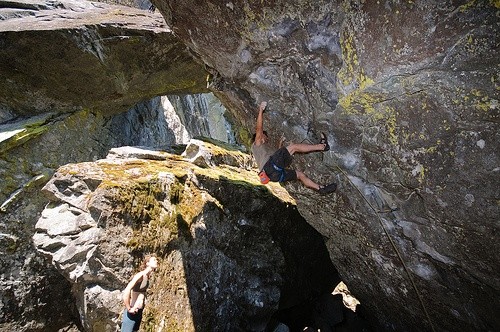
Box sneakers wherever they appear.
[318,183,337,195]
[320,132,330,151]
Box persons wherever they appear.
[249,99,338,196]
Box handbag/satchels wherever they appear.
[258,170,271,184]
